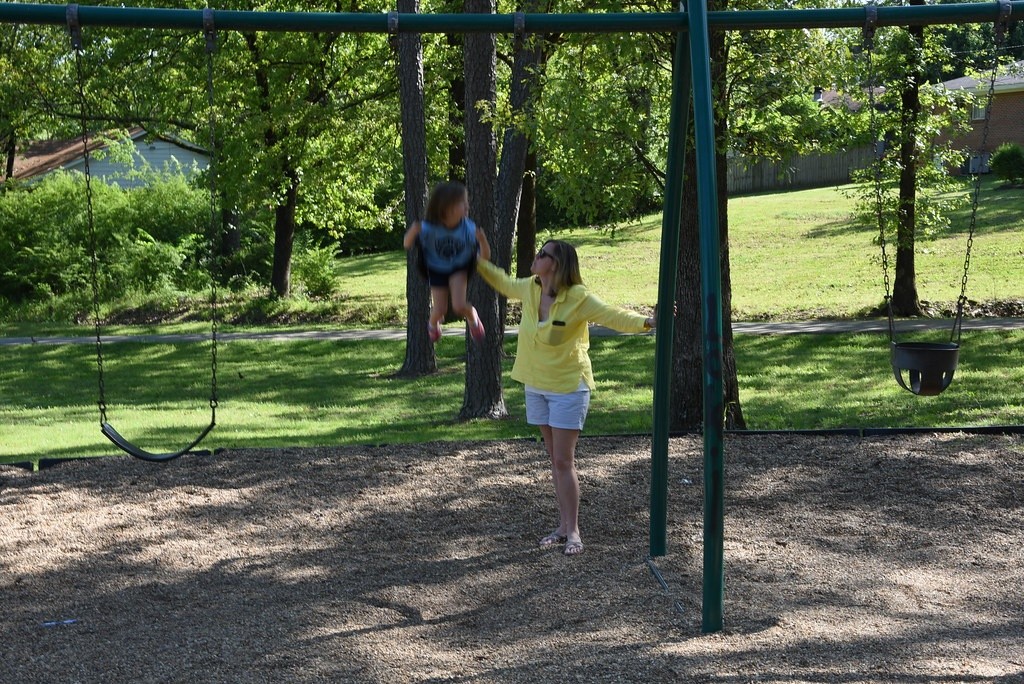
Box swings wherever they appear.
[387,36,528,294]
[72,34,222,463]
[860,21,1010,397]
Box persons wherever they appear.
[404,182,490,343]
[476,239,677,555]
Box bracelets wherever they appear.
[646,318,654,329]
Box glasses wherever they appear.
[539,249,555,263]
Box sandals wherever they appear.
[564,529,585,557]
[539,531,567,546]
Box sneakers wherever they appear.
[428,320,442,343]
[466,306,485,345]
